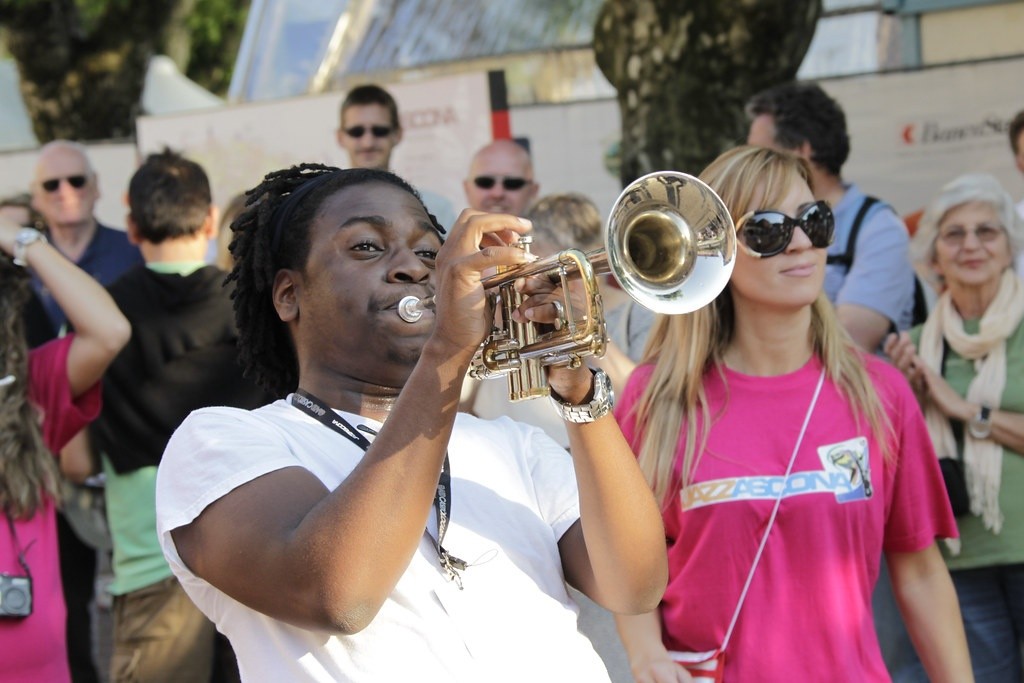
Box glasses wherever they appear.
[343,126,395,138]
[933,226,1004,246]
[731,201,837,259]
[470,176,532,191]
[42,176,90,192]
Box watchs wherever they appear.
[13,227,47,267]
[550,367,615,423]
[969,406,992,439]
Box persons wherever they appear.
[463,142,625,318]
[30,140,145,683]
[59,151,278,683]
[883,174,1024,683]
[156,163,669,683]
[615,144,974,683]
[1006,111,1024,279]
[339,85,403,171]
[744,84,915,362]
[0,207,132,683]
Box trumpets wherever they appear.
[398,170,738,407]
[489,192,603,258]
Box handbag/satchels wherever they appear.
[932,456,972,520]
